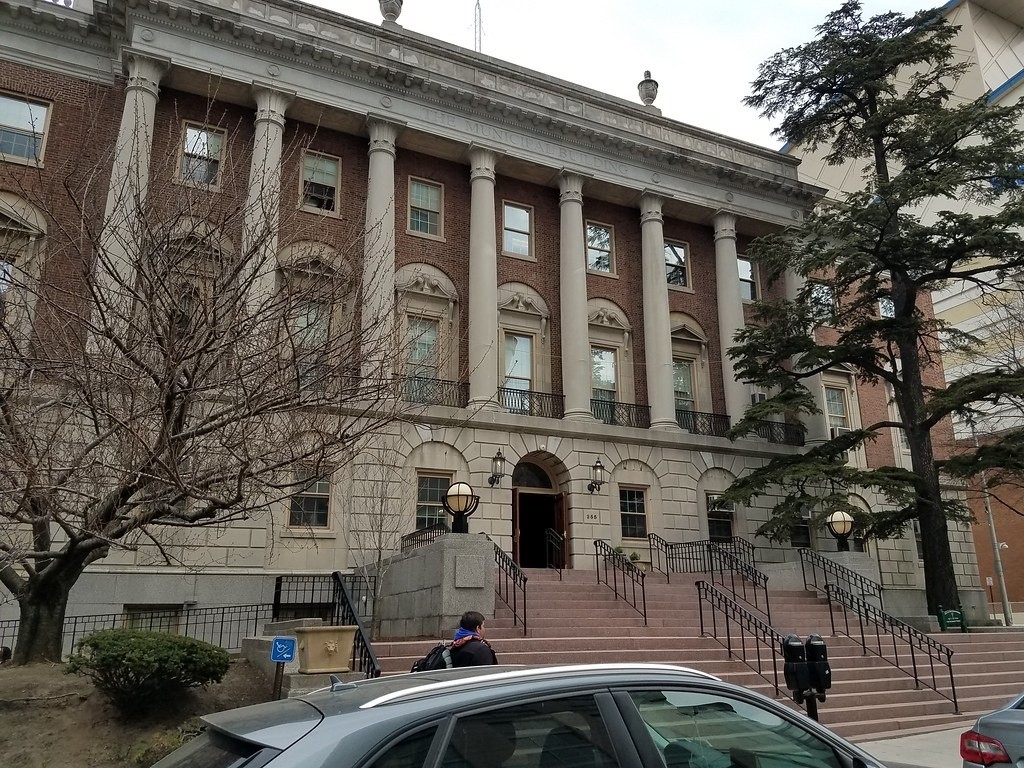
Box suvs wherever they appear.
[140,658,894,768]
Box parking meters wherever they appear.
[783,632,832,724]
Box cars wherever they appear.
[959,689,1024,768]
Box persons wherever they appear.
[449,611,499,667]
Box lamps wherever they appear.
[488,448,504,485]
[587,458,604,492]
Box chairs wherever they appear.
[542,727,598,768]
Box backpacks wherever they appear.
[411,639,482,673]
